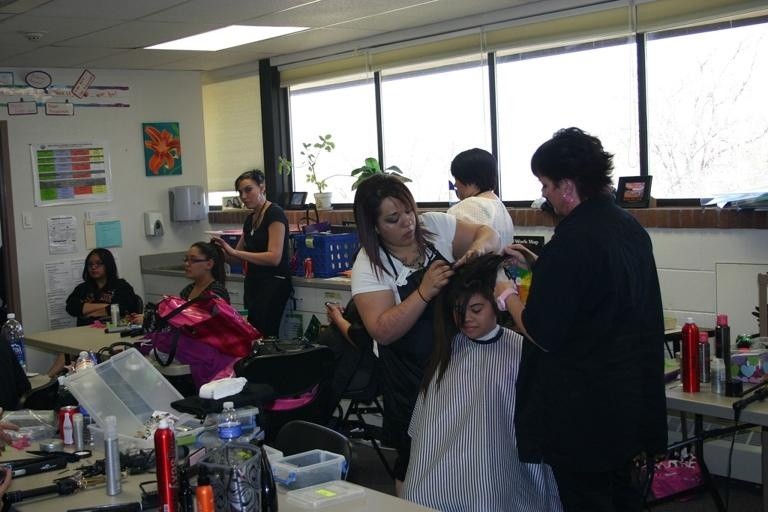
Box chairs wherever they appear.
[273,419,353,481]
[339,368,397,482]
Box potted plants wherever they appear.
[279,134,359,211]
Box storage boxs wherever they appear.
[62,348,197,438]
[89,412,206,460]
[286,481,365,512]
[270,449,347,491]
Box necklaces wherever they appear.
[250,201,267,236]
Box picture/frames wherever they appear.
[615,176,652,208]
[289,191,307,210]
[221,196,244,210]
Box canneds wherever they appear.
[241,260,247,276]
[59,405,80,444]
[304,258,314,279]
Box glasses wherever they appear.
[183,255,209,263]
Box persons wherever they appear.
[180,239,230,306]
[65,248,137,328]
[318,126,668,512]
[211,169,293,338]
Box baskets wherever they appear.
[220,234,249,273]
[289,224,359,280]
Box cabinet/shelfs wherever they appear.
[143,261,351,351]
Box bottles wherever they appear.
[216,401,239,442]
[714,312,732,396]
[62,412,73,444]
[710,355,725,397]
[101,415,122,496]
[194,467,214,511]
[2,312,26,373]
[73,411,84,454]
[153,419,180,512]
[79,381,97,446]
[680,316,701,395]
[696,330,711,385]
[109,303,121,328]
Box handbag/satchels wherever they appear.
[634,442,708,502]
[296,202,331,235]
[233,334,335,400]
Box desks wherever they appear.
[22,324,191,378]
[660,381,768,511]
[663,325,716,359]
